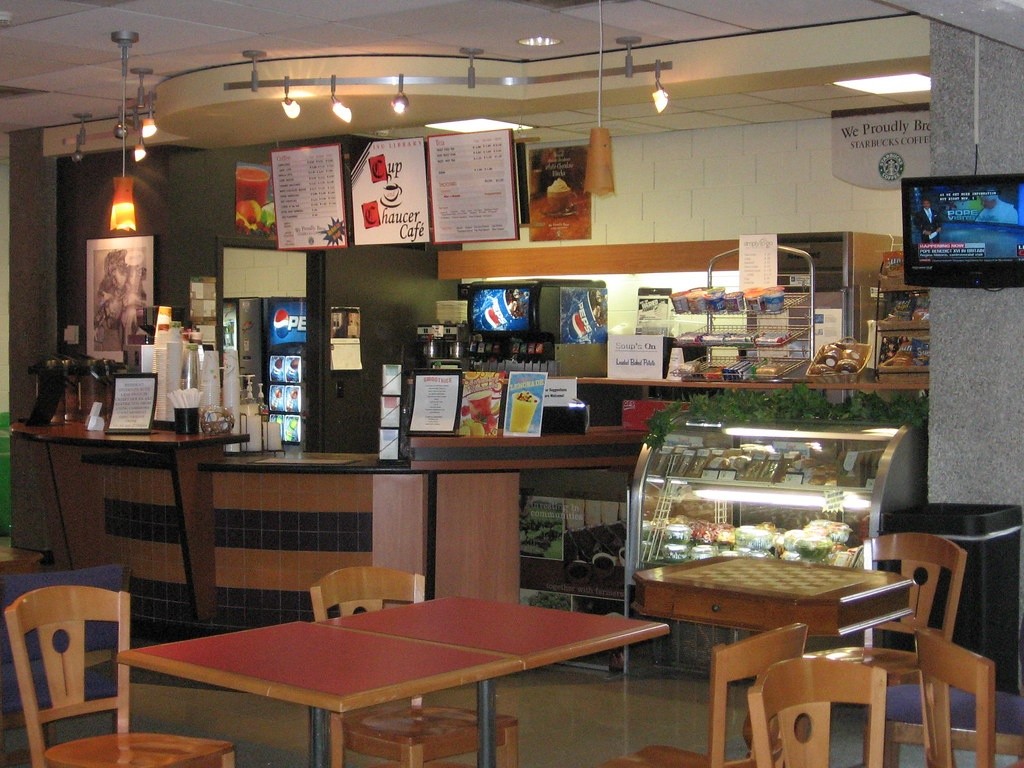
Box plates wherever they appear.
[540,208,577,216]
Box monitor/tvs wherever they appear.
[901,174,1024,289]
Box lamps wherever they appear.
[69,33,163,231]
[584,0,614,199]
[651,62,672,110]
[327,77,351,123]
[391,72,410,115]
[283,77,302,118]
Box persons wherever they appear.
[913,199,944,245]
[974,190,1018,224]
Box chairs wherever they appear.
[0,531,1024,768]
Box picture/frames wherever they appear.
[82,233,158,364]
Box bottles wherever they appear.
[286,418,298,441]
[275,415,282,423]
[271,387,283,410]
[287,358,300,381]
[271,357,284,379]
[287,388,298,411]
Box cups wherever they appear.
[481,290,515,330]
[152,306,241,452]
[174,407,197,433]
[619,547,625,566]
[666,348,684,381]
[566,560,591,585]
[509,393,540,433]
[561,291,603,343]
[236,166,270,207]
[592,552,618,581]
[546,187,577,210]
[467,392,492,417]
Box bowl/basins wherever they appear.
[670,287,788,312]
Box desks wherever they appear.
[633,554,916,766]
[115,619,522,767]
[315,596,670,768]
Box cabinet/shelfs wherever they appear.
[626,415,921,649]
[875,250,936,378]
[668,245,815,381]
